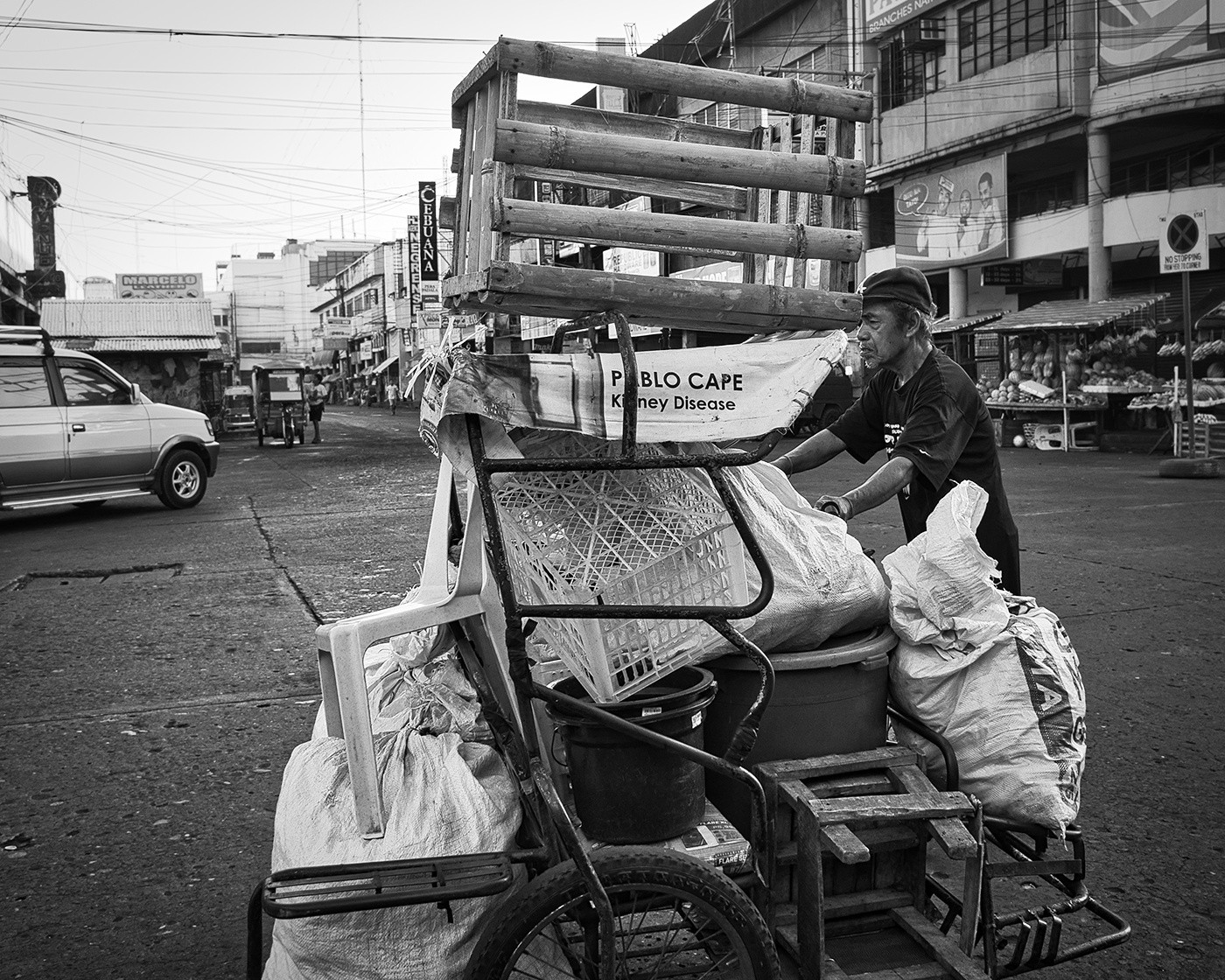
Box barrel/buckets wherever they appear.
[544,663,718,845]
[706,623,900,825]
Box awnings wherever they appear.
[36,337,222,352]
[373,355,399,374]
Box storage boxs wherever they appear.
[1081,384,1127,394]
[1146,385,1162,392]
[498,432,756,704]
[1128,388,1148,393]
[992,418,1026,448]
[1019,380,1055,398]
[1177,421,1225,458]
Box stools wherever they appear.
[747,744,993,980]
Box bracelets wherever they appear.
[842,496,853,519]
[784,455,792,479]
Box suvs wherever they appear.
[0,323,223,517]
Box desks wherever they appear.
[1126,398,1225,455]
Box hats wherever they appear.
[852,267,933,317]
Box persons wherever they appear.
[769,266,1021,615]
[386,379,399,415]
[916,171,1002,257]
[308,374,327,443]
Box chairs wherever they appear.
[1033,421,1100,452]
[312,446,557,841]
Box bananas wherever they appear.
[1157,339,1225,361]
[1068,326,1157,361]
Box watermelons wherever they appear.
[1193,362,1225,401]
[1008,340,1133,388]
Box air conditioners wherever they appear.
[899,17,945,51]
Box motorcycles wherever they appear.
[251,359,310,450]
